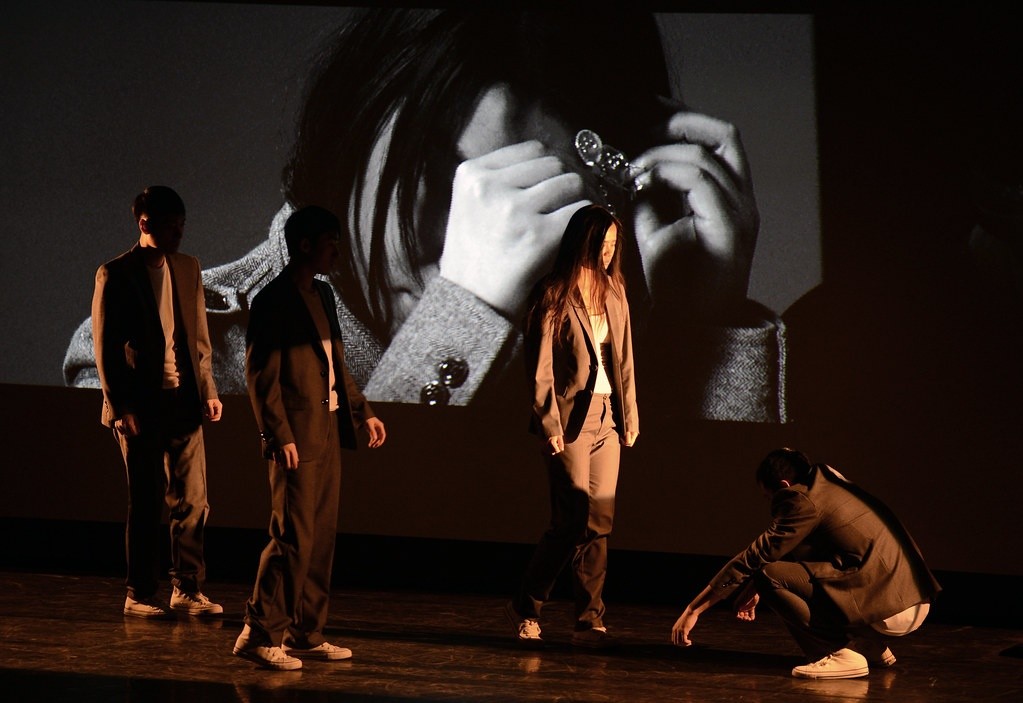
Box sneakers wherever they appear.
[506,599,544,643]
[124,595,169,617]
[847,640,896,668]
[170,586,223,615]
[281,637,352,660]
[791,647,870,679]
[233,622,302,671]
[570,627,625,650]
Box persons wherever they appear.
[92,185,223,618]
[229,206,386,671]
[670,448,941,679]
[61,0,794,425]
[505,205,639,641]
